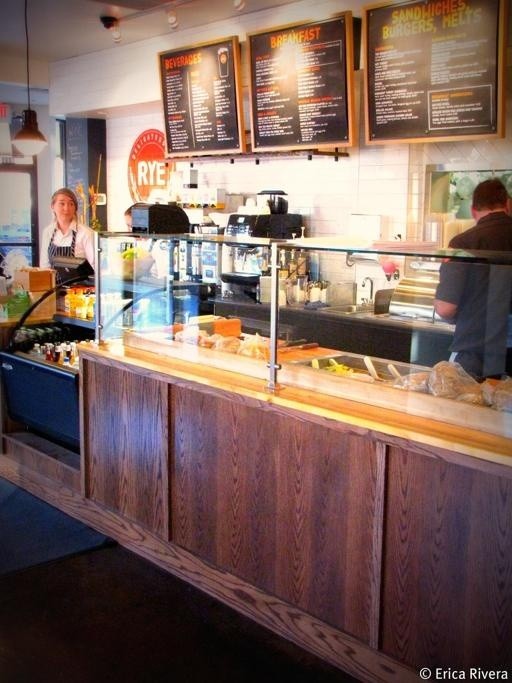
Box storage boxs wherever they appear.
[0,270,55,317]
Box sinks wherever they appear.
[317,304,374,316]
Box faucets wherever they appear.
[362,277,373,304]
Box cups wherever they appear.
[287,275,308,306]
[217,48,230,78]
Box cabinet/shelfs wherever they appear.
[0,318,59,432]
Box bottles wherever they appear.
[14,287,96,368]
[261,248,307,278]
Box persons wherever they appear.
[40,187,95,271]
[124,203,146,232]
[433,179,512,384]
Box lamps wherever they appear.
[110,29,122,46]
[167,10,178,29]
[10,0,49,156]
[233,2,247,11]
